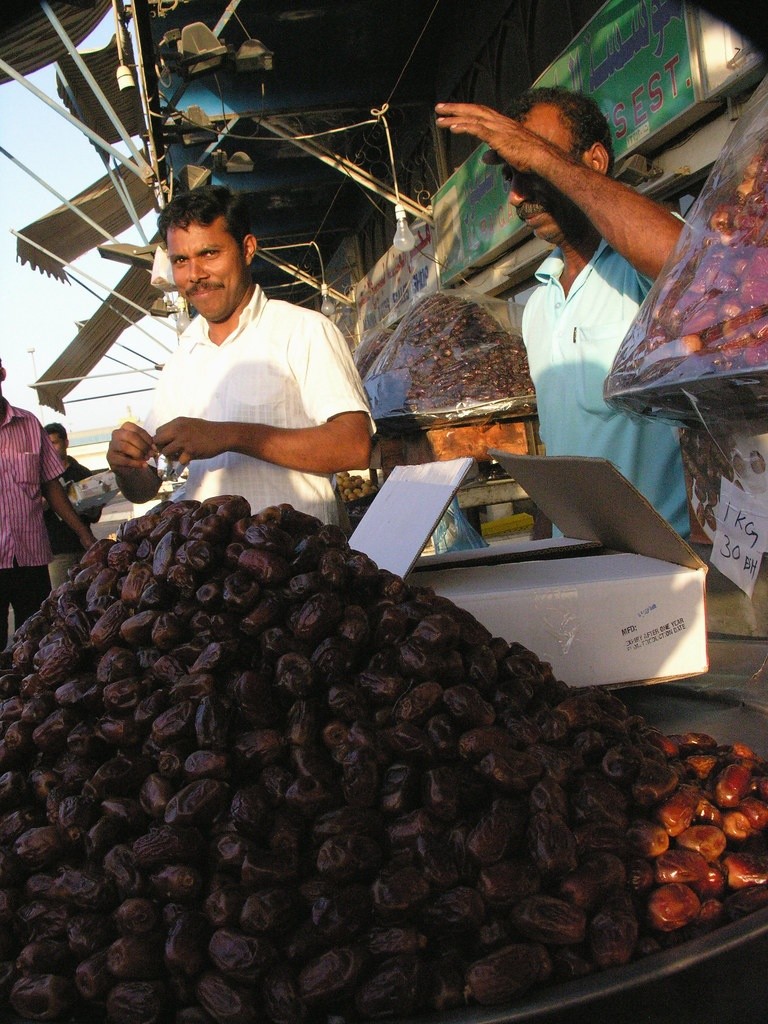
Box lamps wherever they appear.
[176,21,227,83]
[177,163,211,191]
[112,0,136,93]
[150,297,169,318]
[179,104,220,148]
[226,151,254,173]
[97,243,154,271]
[234,38,274,74]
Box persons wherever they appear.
[108,184,381,531]
[0,358,101,657]
[40,422,103,590]
[432,86,688,542]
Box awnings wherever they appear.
[55,33,146,151]
[0,0,159,188]
[12,149,181,357]
[28,225,171,416]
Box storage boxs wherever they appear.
[54,470,120,521]
[347,449,710,691]
